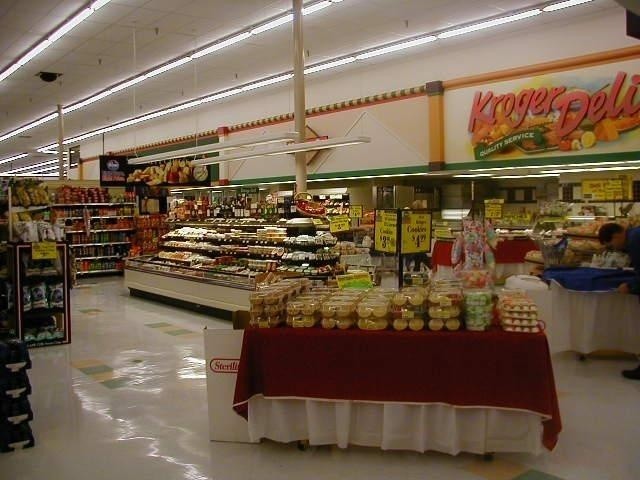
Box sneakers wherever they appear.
[621,366,640,379]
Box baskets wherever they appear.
[296,199,328,218]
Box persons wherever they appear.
[598,224,640,380]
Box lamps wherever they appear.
[190,30,371,167]
[126,20,300,165]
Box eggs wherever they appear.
[499,292,539,332]
